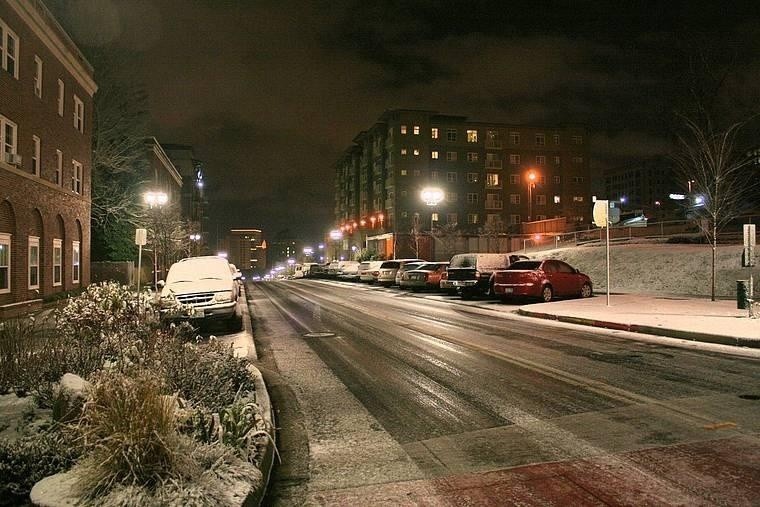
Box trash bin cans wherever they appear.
[746,297,760,318]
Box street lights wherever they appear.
[330,228,344,261]
[143,189,169,295]
[526,168,539,221]
[190,232,202,256]
[419,185,446,261]
[688,178,696,194]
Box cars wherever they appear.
[154,253,246,335]
